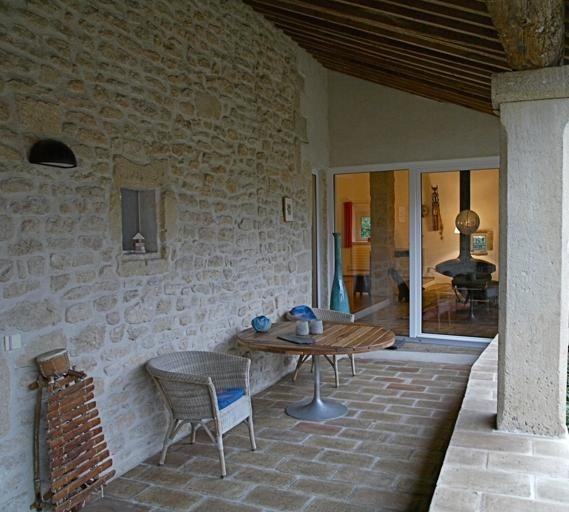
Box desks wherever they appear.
[235,319,396,422]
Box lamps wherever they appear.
[29,138,76,169]
[455,171,480,236]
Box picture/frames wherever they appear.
[283,196,294,223]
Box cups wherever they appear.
[295,320,324,336]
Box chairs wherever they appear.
[285,307,357,388]
[143,350,256,478]
